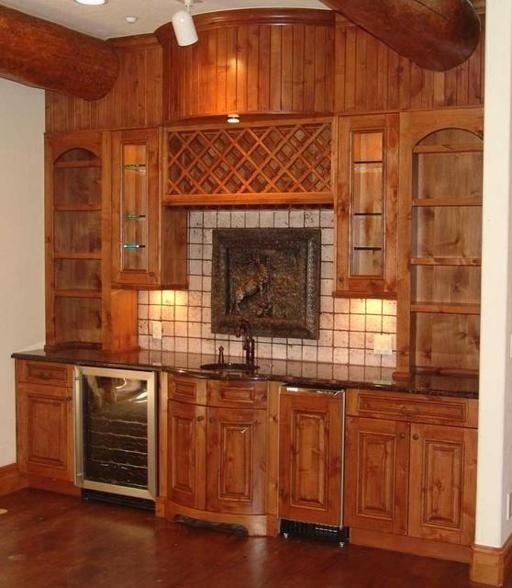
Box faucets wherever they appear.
[235,319,255,367]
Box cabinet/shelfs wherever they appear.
[161,120,334,205]
[157,371,274,536]
[347,388,479,564]
[44,135,138,353]
[14,356,79,496]
[112,128,187,290]
[392,106,486,380]
[275,385,346,531]
[334,113,399,300]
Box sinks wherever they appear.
[200,363,259,370]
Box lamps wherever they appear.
[171,0,202,47]
